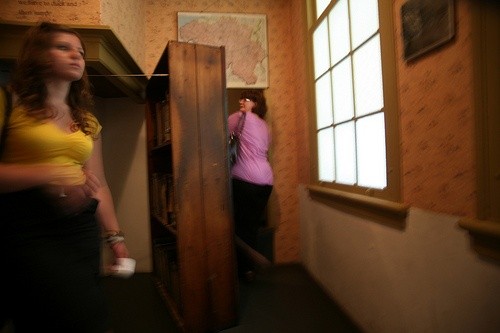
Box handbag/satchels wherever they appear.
[229,112,247,163]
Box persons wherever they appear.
[227,91,273,287]
[0,23,129,333]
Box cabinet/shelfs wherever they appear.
[145,40,242,333]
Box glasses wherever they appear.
[238,98,252,102]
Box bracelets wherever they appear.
[105,232,126,248]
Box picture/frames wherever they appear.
[399,0,458,66]
